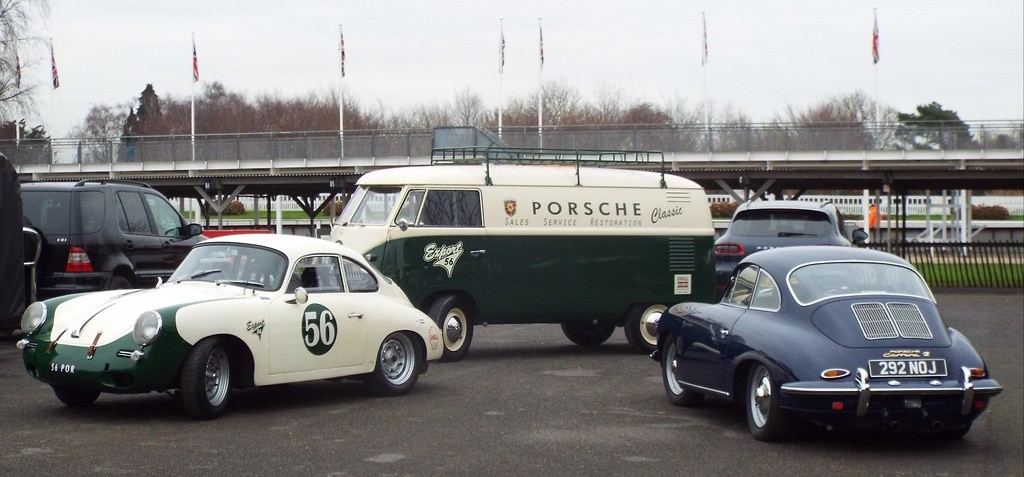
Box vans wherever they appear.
[323,144,717,362]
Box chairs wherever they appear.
[750,213,772,235]
[801,220,828,235]
[300,267,329,287]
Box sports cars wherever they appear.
[649,243,1005,441]
[16,231,446,419]
[714,198,872,301]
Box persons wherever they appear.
[869,203,883,242]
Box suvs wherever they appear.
[19,176,211,302]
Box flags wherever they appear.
[872,8,880,64]
[537,18,545,67]
[339,26,346,80]
[50,35,59,90]
[702,11,709,66]
[192,32,199,85]
[499,20,507,73]
